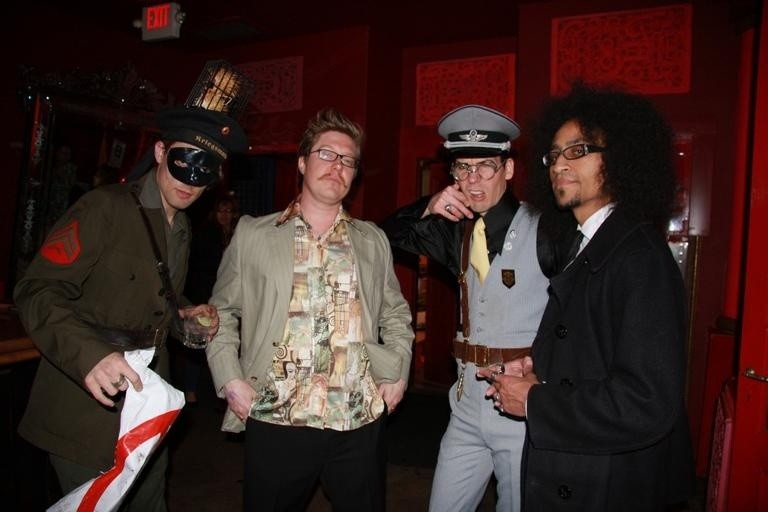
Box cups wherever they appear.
[183,307,214,349]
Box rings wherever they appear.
[496,392,500,401]
[496,363,505,375]
[110,374,126,389]
[490,371,497,381]
[444,204,453,212]
[499,403,504,413]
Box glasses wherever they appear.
[448,159,506,181]
[541,143,604,167]
[308,148,360,169]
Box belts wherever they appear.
[452,340,532,367]
[80,319,173,349]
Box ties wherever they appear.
[564,230,584,267]
[468,217,490,282]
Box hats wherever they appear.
[436,103,521,156]
[153,107,246,163]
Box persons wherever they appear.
[373,102,550,512]
[201,111,416,512]
[474,75,700,511]
[12,105,253,511]
[166,192,245,442]
